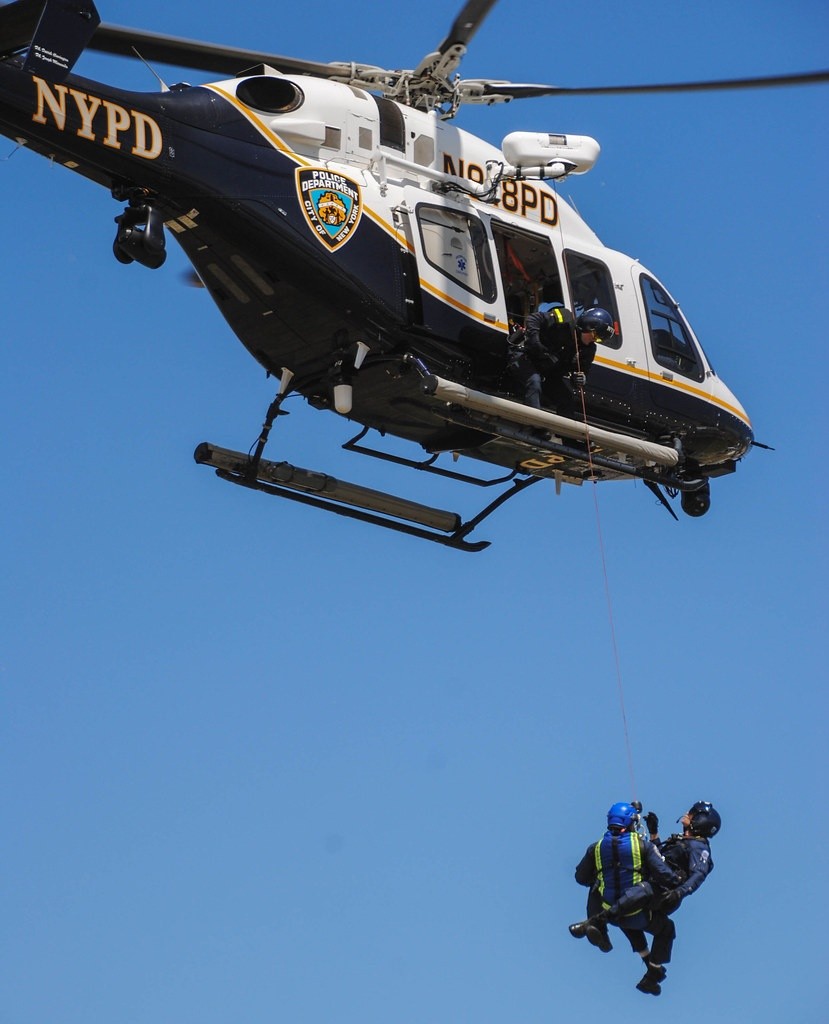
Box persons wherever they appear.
[568,800,721,996]
[509,302,616,410]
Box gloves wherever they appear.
[643,811,658,834]
[659,885,679,909]
[570,372,586,386]
[536,343,549,361]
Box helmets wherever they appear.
[607,803,638,830]
[577,308,614,340]
[692,802,721,838]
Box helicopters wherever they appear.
[0,1,829,555]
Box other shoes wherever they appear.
[658,966,667,982]
[636,976,661,996]
[586,925,613,952]
[569,920,592,938]
